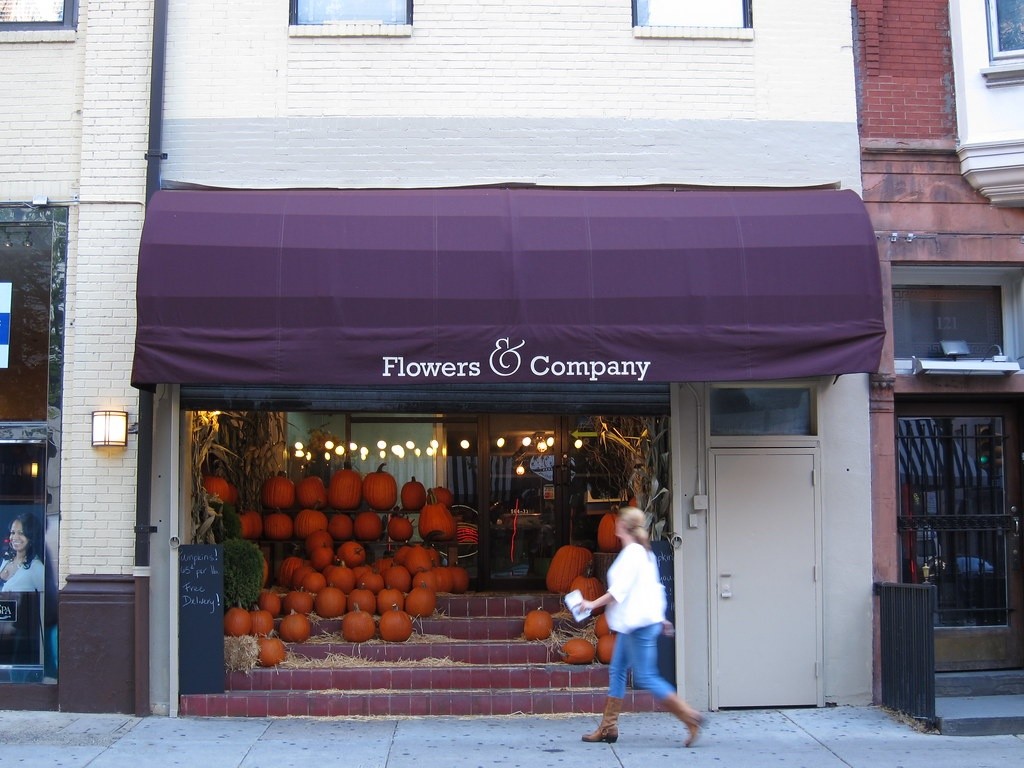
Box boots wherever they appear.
[662,693,707,747]
[582,696,623,742]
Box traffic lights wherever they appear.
[992,431,1005,468]
[977,424,994,469]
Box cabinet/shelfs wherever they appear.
[256,507,461,577]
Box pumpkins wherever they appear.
[557,613,617,664]
[204,460,469,666]
[545,542,605,617]
[524,606,554,641]
[597,510,623,553]
[630,497,636,507]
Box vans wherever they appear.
[915,529,941,565]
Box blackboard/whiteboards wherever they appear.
[178,545,226,704]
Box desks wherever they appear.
[502,513,543,557]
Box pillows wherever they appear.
[456,528,478,543]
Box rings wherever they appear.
[582,606,584,608]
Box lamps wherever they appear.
[980,343,1008,363]
[942,339,971,361]
[91,411,128,446]
[31,463,38,478]
[534,432,548,452]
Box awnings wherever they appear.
[130,186,887,384]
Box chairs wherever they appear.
[449,505,480,559]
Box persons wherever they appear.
[0,512,44,592]
[580,507,706,748]
